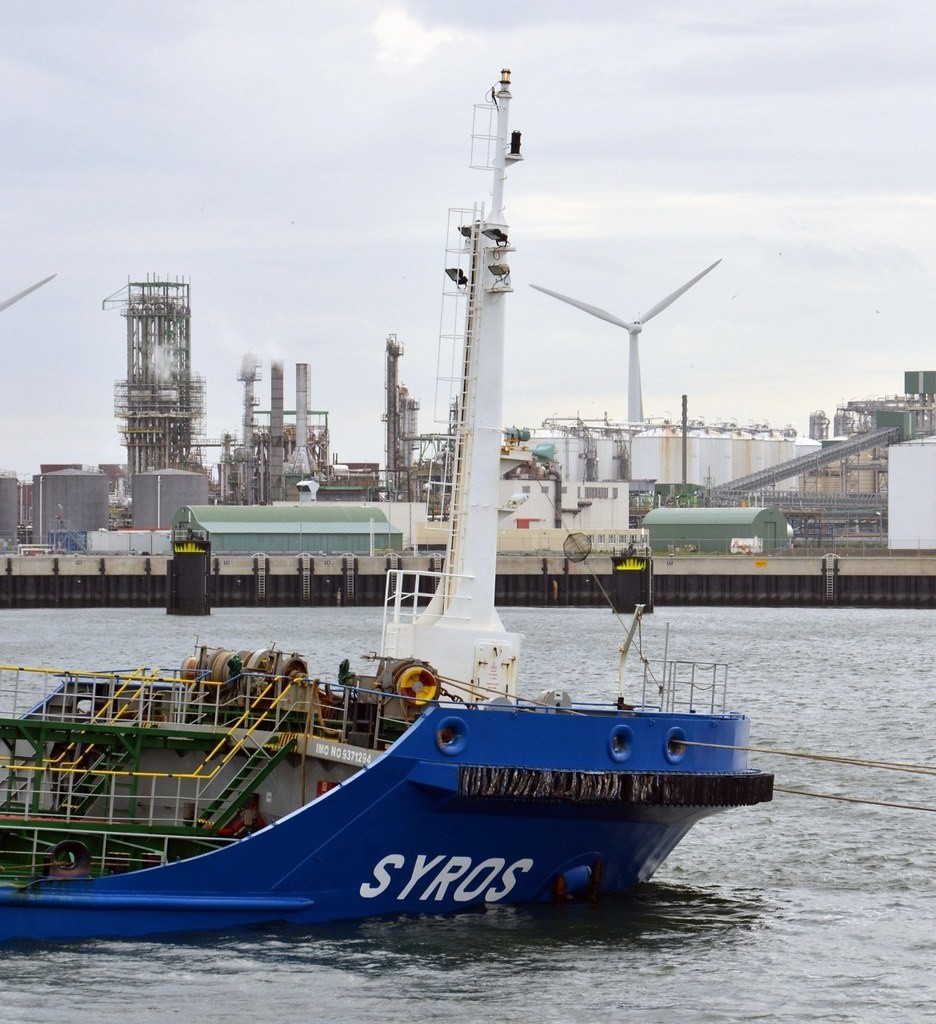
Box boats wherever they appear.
[0,70,775,945]
